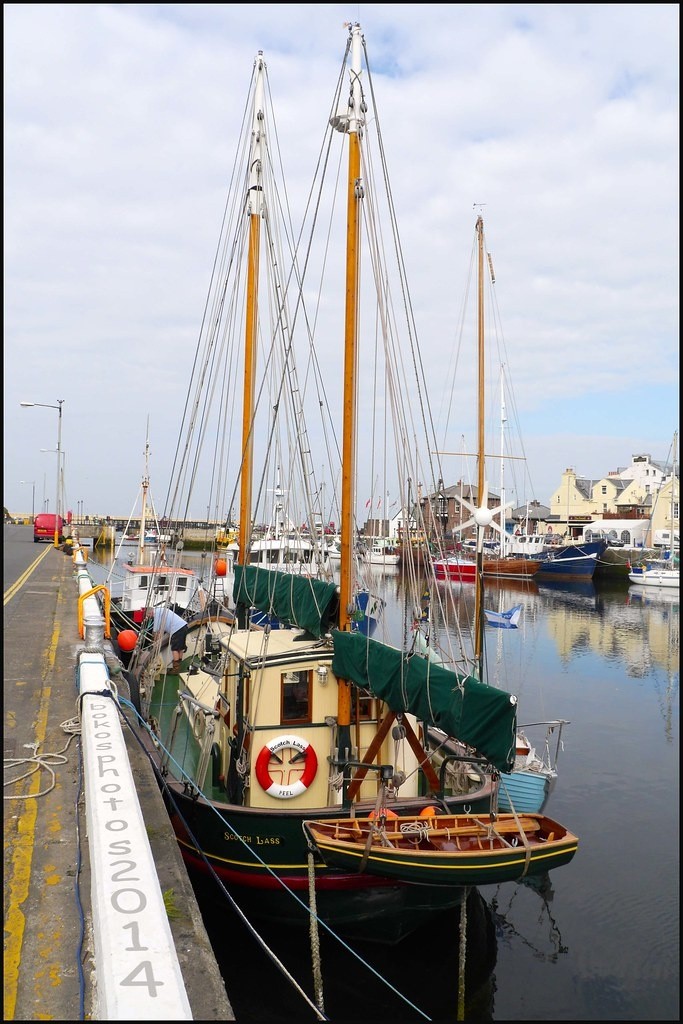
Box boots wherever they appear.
[167,658,182,675]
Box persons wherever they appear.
[145,606,189,675]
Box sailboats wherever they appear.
[86,19,683,894]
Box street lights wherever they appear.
[20,399,64,549]
[40,447,64,528]
[19,480,35,524]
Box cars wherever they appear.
[33,513,66,544]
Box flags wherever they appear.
[484,605,522,629]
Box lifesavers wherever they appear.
[256,735,320,800]
